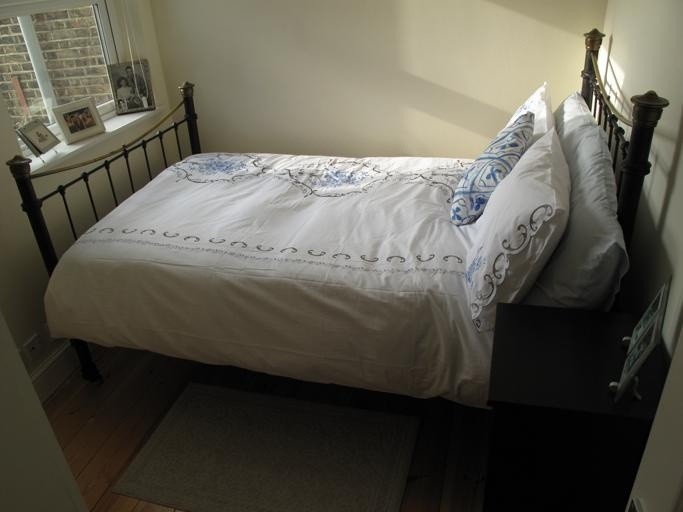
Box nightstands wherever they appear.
[486,299,672,512]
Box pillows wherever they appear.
[461,123,574,337]
[451,108,535,227]
[496,80,555,143]
[541,123,629,308]
[554,88,598,144]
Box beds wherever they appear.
[7,28,670,397]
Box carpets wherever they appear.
[112,381,422,512]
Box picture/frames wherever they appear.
[606,285,670,396]
[16,56,156,156]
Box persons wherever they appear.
[116,77,132,99]
[118,98,127,111]
[128,97,143,109]
[125,65,151,106]
